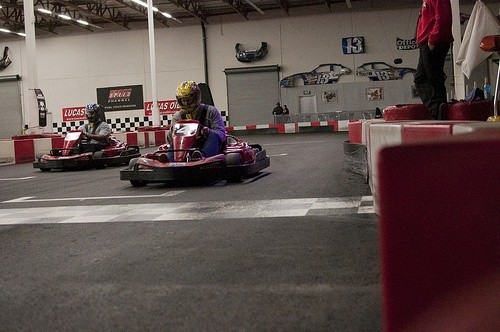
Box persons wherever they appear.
[159,81,227,161]
[272,102,283,115]
[52,104,110,156]
[414,0,454,120]
[282,105,289,116]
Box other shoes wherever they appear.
[159,154,169,163]
[52,148,60,156]
[192,150,202,161]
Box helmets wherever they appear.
[175,81,202,114]
[85,103,100,123]
